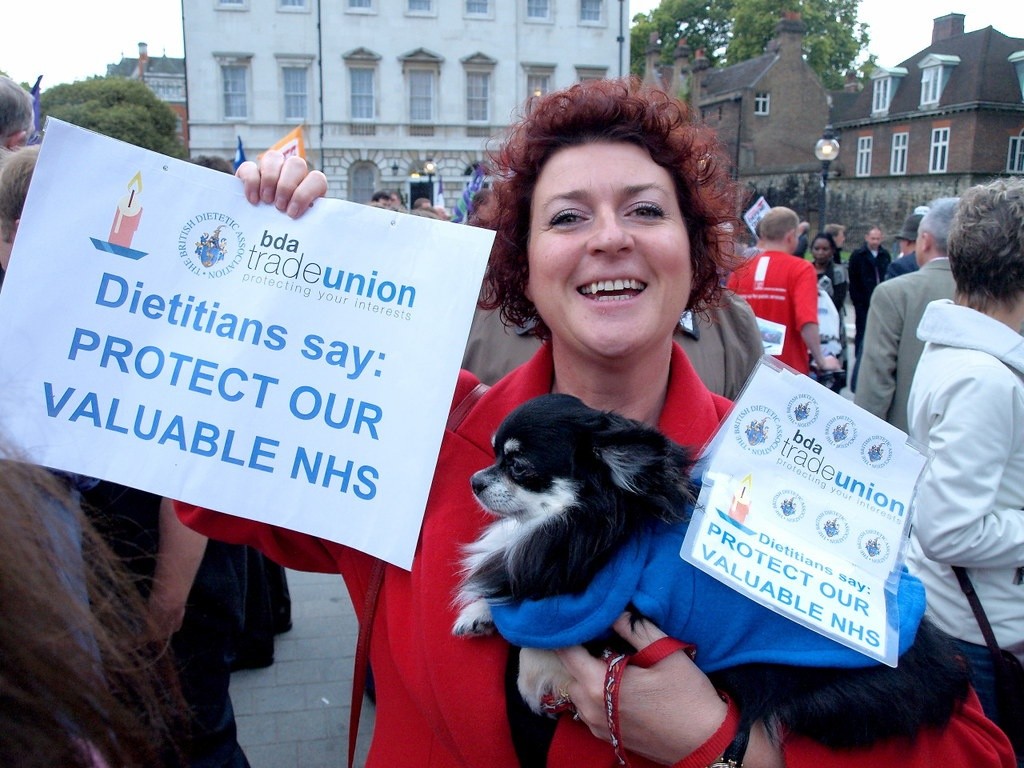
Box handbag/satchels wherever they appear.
[992,648,1024,763]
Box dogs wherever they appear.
[449,391,973,755]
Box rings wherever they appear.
[559,677,573,704]
[572,712,582,722]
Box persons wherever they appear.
[847,225,893,395]
[0,148,257,767]
[724,207,844,378]
[885,212,926,285]
[168,74,1017,768]
[454,277,760,402]
[716,217,845,335]
[904,175,1023,768]
[2,73,37,150]
[856,195,963,438]
[362,186,501,228]
[181,151,300,671]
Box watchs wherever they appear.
[706,707,748,768]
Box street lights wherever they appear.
[812,123,840,238]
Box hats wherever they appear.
[893,214,924,242]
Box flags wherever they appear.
[231,135,249,170]
[258,120,307,161]
[26,75,44,145]
[453,163,484,223]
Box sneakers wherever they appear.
[223,650,275,672]
